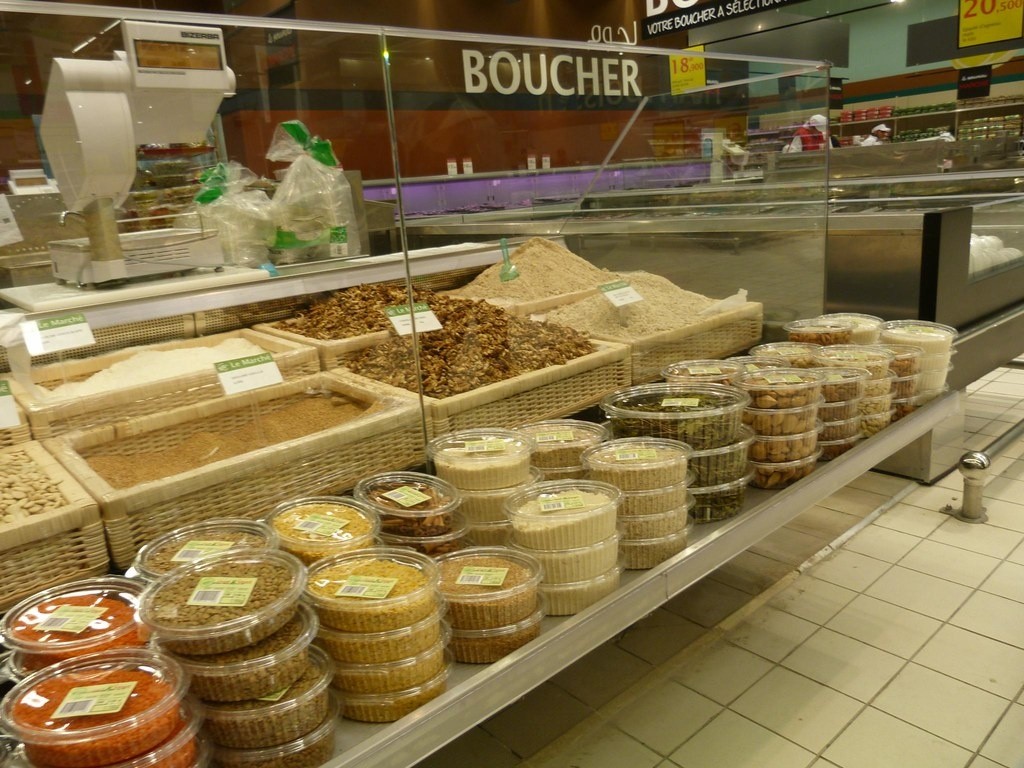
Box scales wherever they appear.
[722,138,763,179]
[38,19,237,288]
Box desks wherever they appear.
[0,265,268,312]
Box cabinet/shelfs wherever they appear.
[741,96,1024,169]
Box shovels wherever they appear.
[500,238,520,281]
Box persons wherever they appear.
[861,124,892,146]
[782,115,833,152]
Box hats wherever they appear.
[810,115,827,127]
[872,124,891,133]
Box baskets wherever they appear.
[0,272,762,601]
